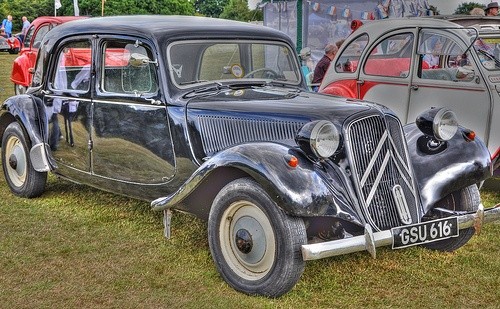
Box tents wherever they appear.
[258,0,438,92]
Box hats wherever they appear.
[484,2,500,11]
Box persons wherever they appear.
[1,15,13,38]
[22,16,30,36]
[298,3,500,79]
[312,42,338,92]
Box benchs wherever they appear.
[346,58,430,76]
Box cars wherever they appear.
[317,16,500,171]
[10,15,132,96]
[0,13,500,299]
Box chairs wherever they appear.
[171,47,190,84]
